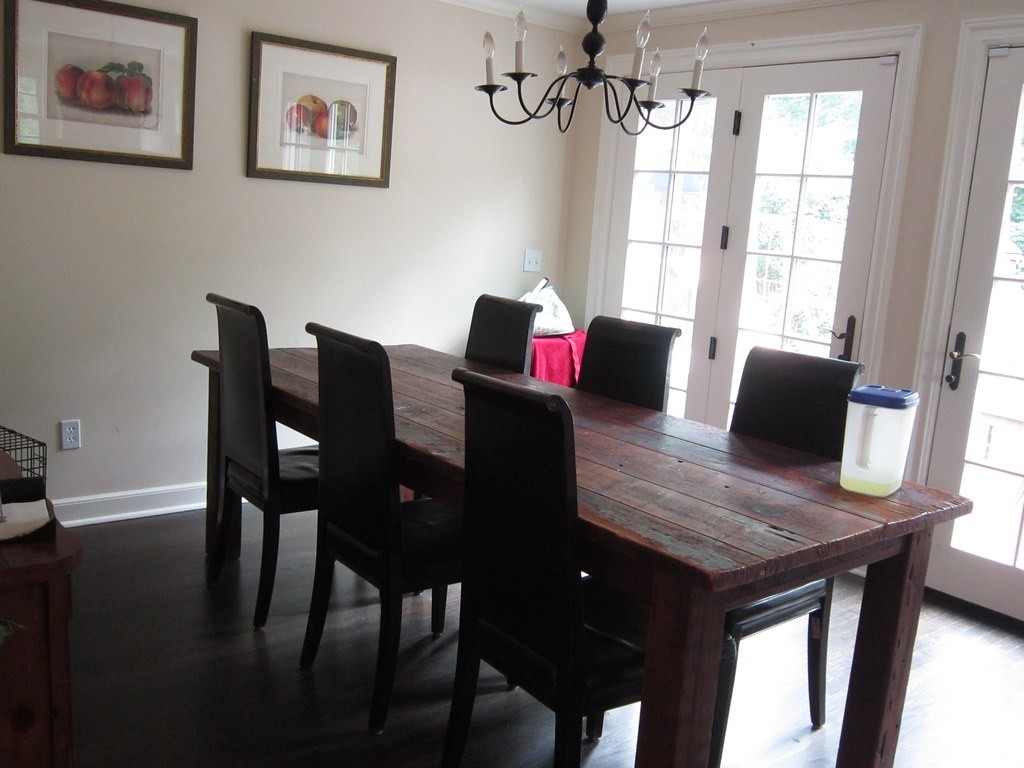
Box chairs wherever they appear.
[440,369,739,768]
[464,294,543,376]
[299,321,462,736]
[575,315,682,414]
[205,293,322,630]
[724,346,866,727]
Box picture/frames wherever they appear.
[4,0,199,169]
[246,31,398,189]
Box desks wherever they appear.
[191,343,975,767]
[0,516,85,768]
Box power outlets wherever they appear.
[60,419,81,450]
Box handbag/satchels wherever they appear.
[520,278,575,336]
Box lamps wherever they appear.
[474,0,713,135]
